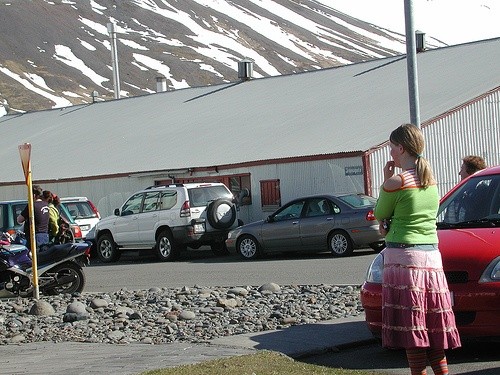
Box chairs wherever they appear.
[308,203,322,216]
[322,200,335,214]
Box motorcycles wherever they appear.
[0,224,93,298]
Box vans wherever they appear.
[0,197,101,240]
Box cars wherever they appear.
[359,164,500,337]
[224,192,388,260]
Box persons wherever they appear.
[458,155,490,222]
[17,186,49,249]
[373,123,461,375]
[42,190,60,244]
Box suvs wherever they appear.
[96,182,239,260]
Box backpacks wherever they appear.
[49,207,75,244]
